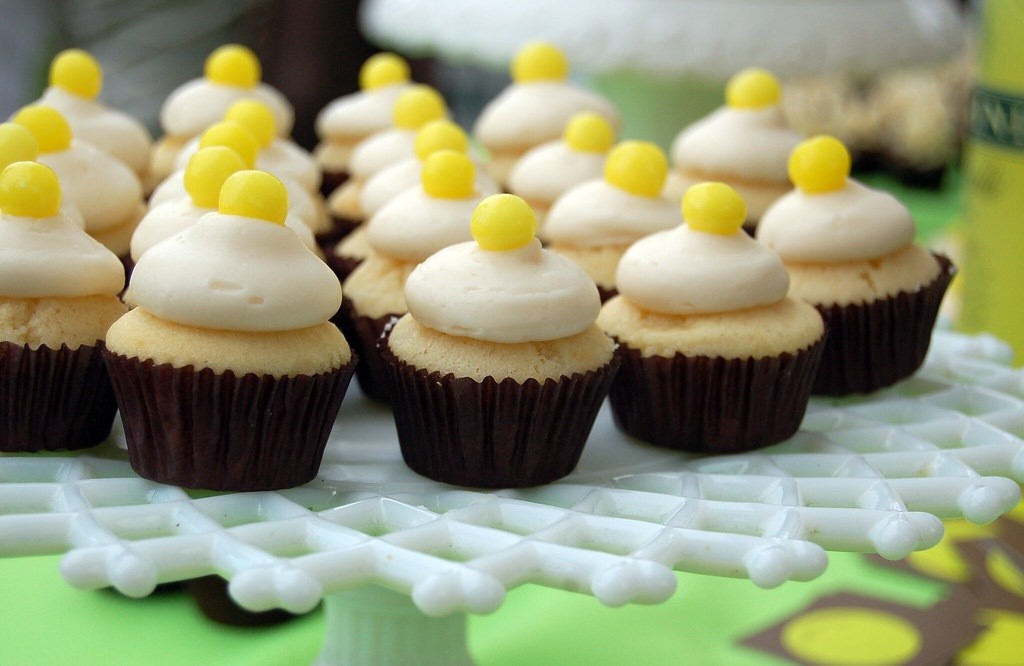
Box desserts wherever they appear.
[0,48,955,489]
